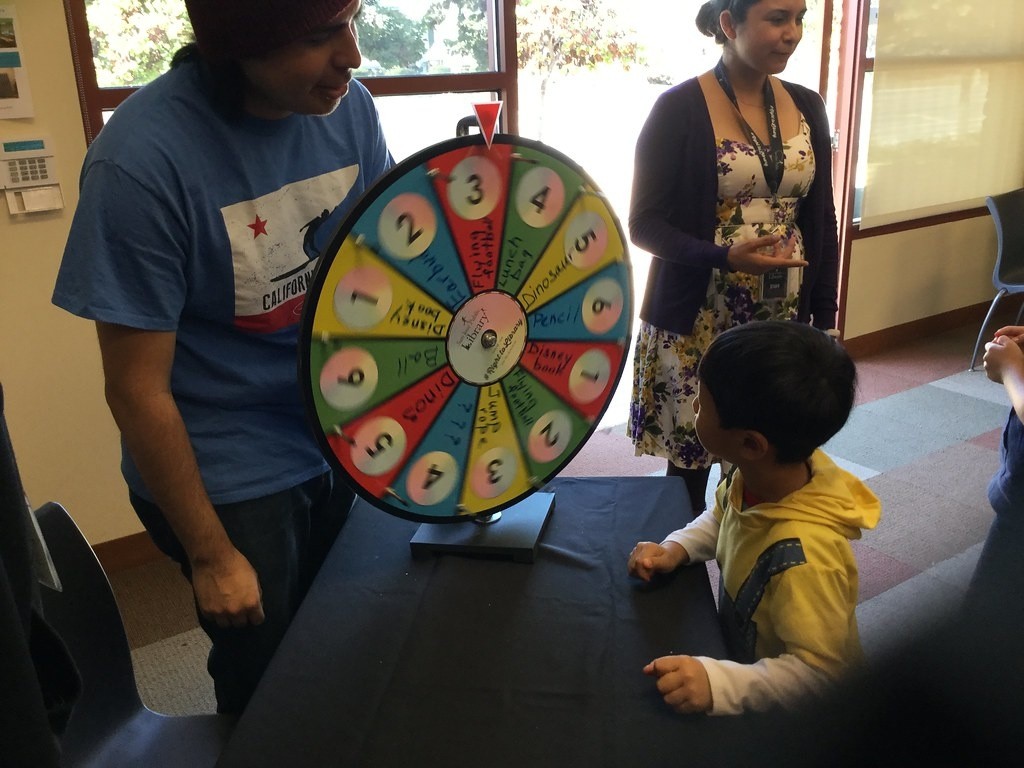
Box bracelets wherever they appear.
[822,328,841,339]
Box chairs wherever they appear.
[968,187,1024,373]
[0,499,241,767]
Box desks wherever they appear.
[217,475,748,768]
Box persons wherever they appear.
[962,325,1023,635]
[47,0,403,744]
[628,0,844,518]
[627,318,884,721]
